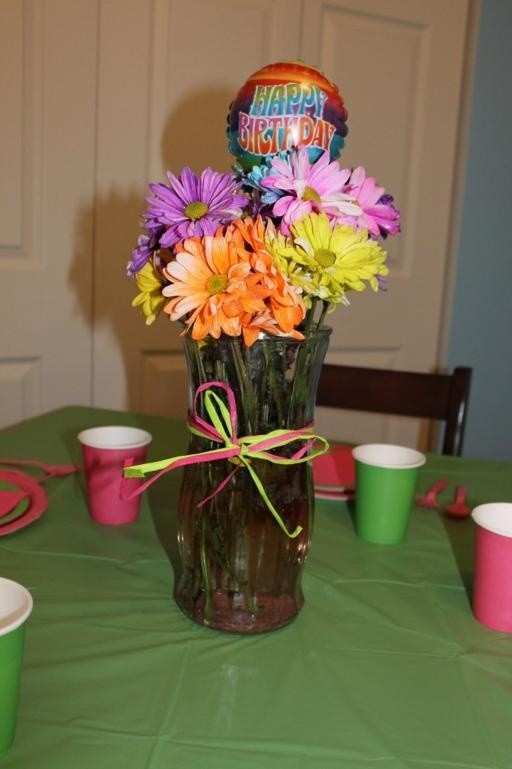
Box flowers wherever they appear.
[124,144,402,628]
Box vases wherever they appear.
[173,325,334,636]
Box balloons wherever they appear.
[225,61,349,167]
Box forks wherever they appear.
[415,478,449,510]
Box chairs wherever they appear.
[317,365,473,457]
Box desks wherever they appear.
[1,405,512,768]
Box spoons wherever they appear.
[445,484,472,519]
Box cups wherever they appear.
[468,500,512,636]
[0,575,35,762]
[76,425,155,527]
[351,442,428,548]
